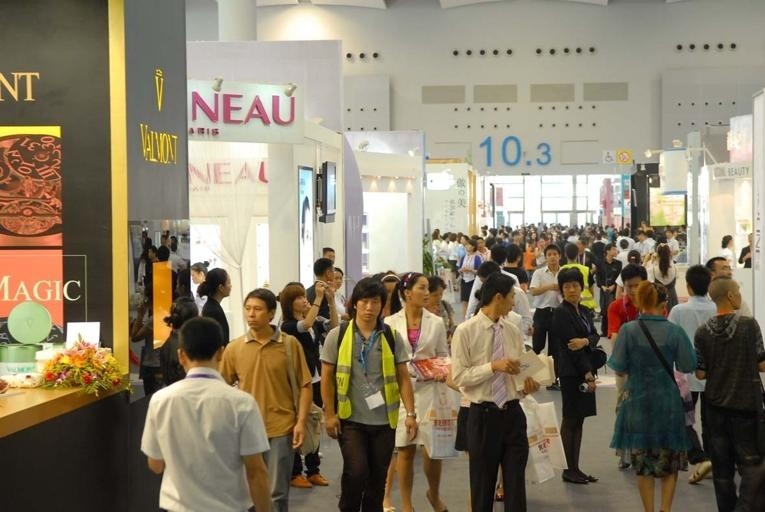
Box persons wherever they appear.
[602,264,669,390]
[323,247,335,265]
[595,243,622,337]
[705,257,753,316]
[669,265,720,483]
[475,262,535,501]
[440,232,449,264]
[275,282,305,302]
[547,265,607,485]
[530,243,566,391]
[694,274,765,512]
[451,273,542,512]
[333,266,351,322]
[423,274,458,344]
[479,221,553,249]
[191,261,209,286]
[632,230,655,255]
[159,297,201,385]
[615,250,642,298]
[320,277,418,512]
[575,234,599,296]
[432,230,442,258]
[739,232,753,268]
[219,288,334,512]
[554,221,633,252]
[377,272,401,512]
[139,318,274,511]
[616,229,635,252]
[719,234,735,269]
[666,230,681,263]
[276,283,338,488]
[476,237,492,261]
[197,269,233,358]
[448,233,459,269]
[465,243,521,322]
[384,272,451,509]
[646,242,678,311]
[564,242,598,319]
[606,279,699,512]
[134,230,190,393]
[455,235,469,266]
[302,258,337,345]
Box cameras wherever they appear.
[315,280,328,289]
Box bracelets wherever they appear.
[585,376,593,382]
[328,303,336,309]
[313,302,321,309]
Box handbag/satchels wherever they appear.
[637,318,696,428]
[522,397,568,485]
[282,333,327,457]
[418,378,461,459]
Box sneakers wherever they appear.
[688,460,712,484]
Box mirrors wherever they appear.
[127,219,191,406]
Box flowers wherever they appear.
[40,341,135,398]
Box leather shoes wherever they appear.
[580,472,599,482]
[425,489,449,512]
[383,504,396,512]
[308,473,330,486]
[289,476,313,488]
[496,487,504,501]
[562,470,589,484]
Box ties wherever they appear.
[490,324,508,409]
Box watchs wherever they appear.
[405,412,417,417]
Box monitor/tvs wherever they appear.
[314,162,337,224]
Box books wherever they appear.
[409,358,452,380]
